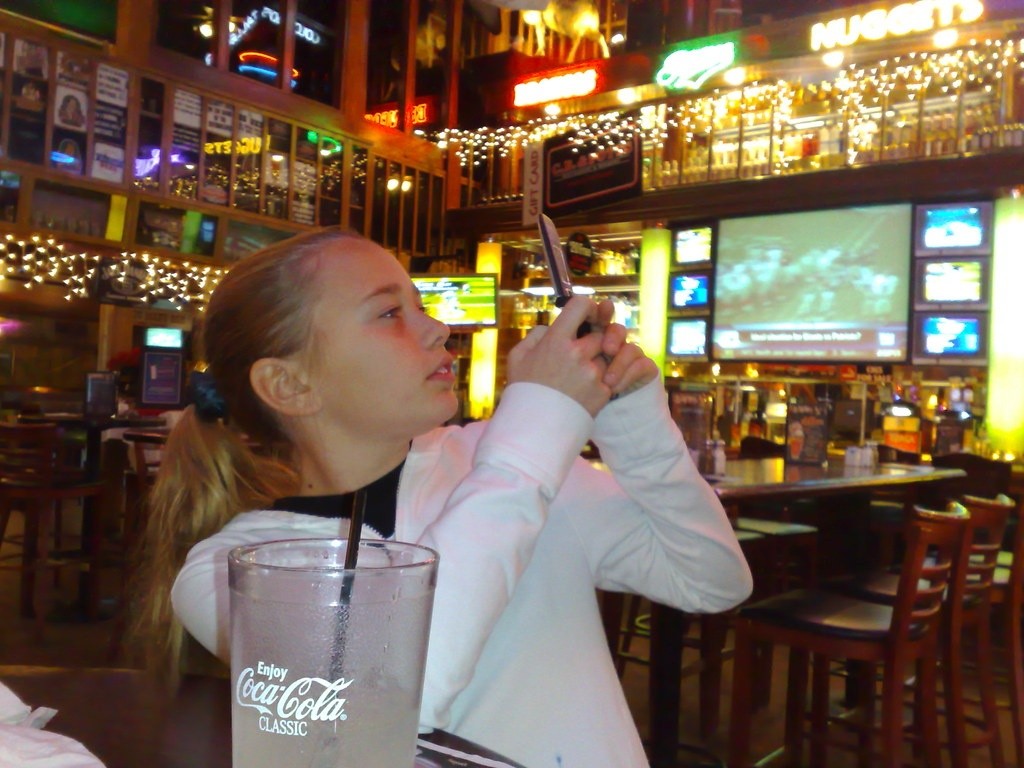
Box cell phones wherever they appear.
[537,213,591,340]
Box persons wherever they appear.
[137,226,753,768]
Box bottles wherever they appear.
[881,384,920,464]
[717,385,833,463]
[699,439,727,482]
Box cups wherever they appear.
[228,539,439,768]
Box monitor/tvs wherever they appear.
[662,199,988,366]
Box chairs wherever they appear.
[0,395,1024,768]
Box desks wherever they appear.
[0,670,527,768]
[602,448,969,768]
[16,415,167,624]
